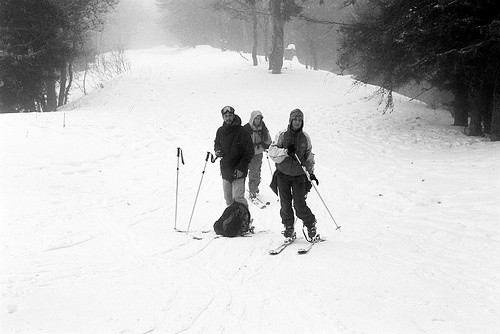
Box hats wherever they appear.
[289,108,304,126]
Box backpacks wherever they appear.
[214,202,248,238]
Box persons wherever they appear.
[268,109,319,241]
[243,110,272,202]
[214,106,255,208]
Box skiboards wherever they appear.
[245,189,270,209]
[269,234,321,254]
[193,218,271,240]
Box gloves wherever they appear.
[288,144,296,155]
[310,174,318,185]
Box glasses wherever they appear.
[221,106,234,114]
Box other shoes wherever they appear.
[285,225,294,238]
[249,189,258,198]
[307,223,316,237]
